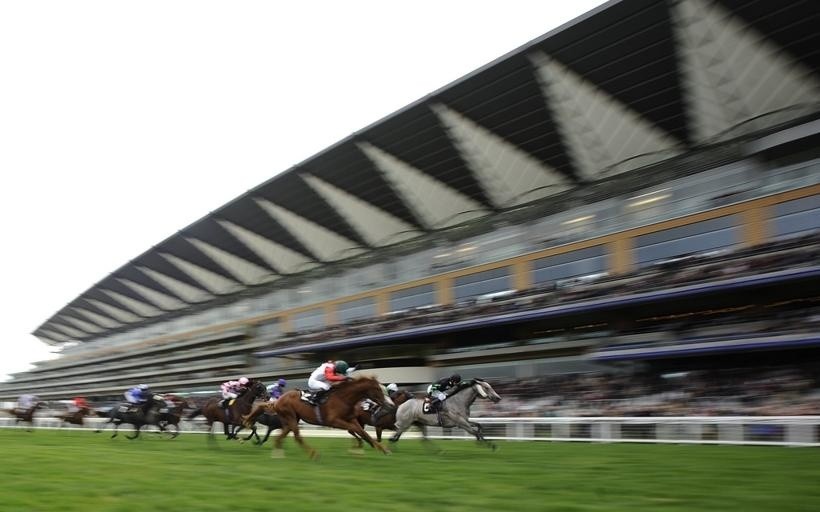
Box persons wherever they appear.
[219,377,252,408]
[265,378,288,401]
[421,374,464,415]
[365,381,398,415]
[305,359,355,406]
[122,383,151,409]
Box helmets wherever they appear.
[279,379,286,387]
[334,361,348,374]
[386,384,398,392]
[239,377,249,385]
[451,375,461,383]
[140,384,148,390]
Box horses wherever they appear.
[388,378,502,452]
[187,374,427,462]
[95,395,196,439]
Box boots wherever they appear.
[426,399,440,409]
[309,388,324,406]
